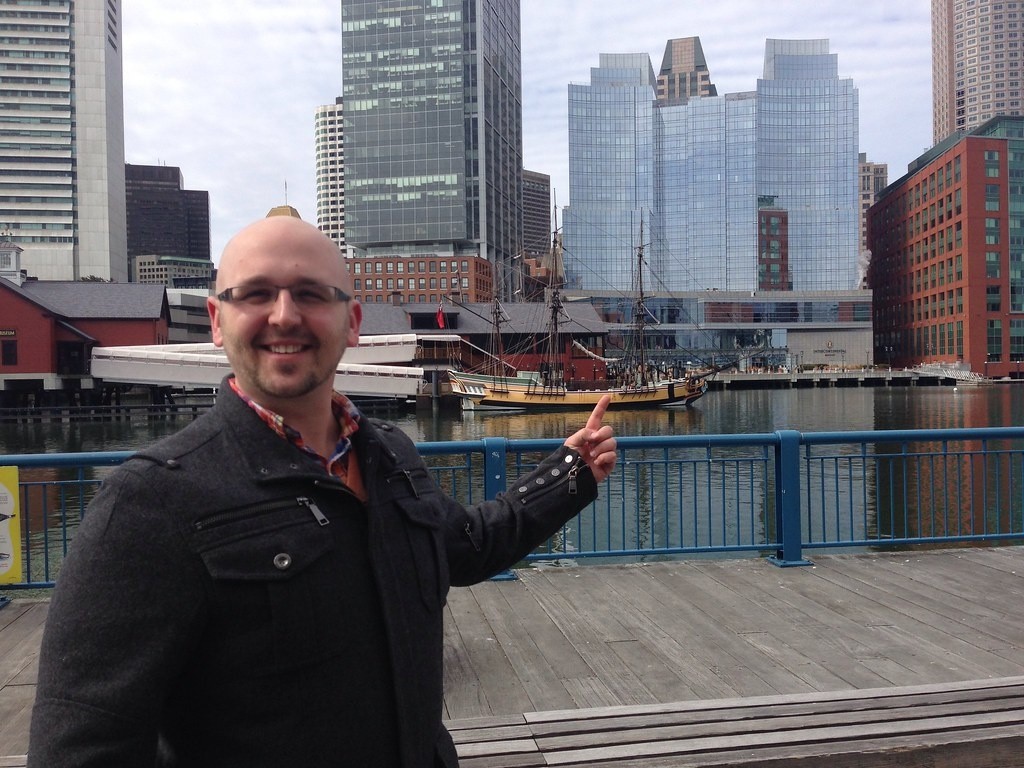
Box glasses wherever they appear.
[217,286,351,309]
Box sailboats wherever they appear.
[443,188,766,409]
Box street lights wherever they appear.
[885,346,893,372]
[790,353,793,374]
[742,350,749,374]
[866,351,869,373]
[927,344,933,363]
[841,349,846,373]
[800,351,804,373]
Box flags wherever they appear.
[436,299,445,329]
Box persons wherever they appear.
[29,213,619,768]
